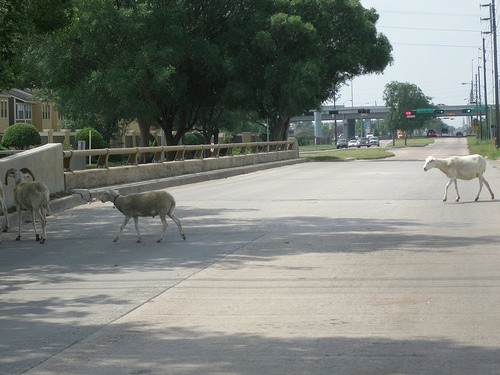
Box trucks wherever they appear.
[441,128,449,136]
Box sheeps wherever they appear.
[0,168,51,245]
[423,153,494,202]
[99,189,186,243]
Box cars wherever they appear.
[427,130,436,137]
[367,136,379,147]
[358,138,370,148]
[337,139,348,149]
[366,135,373,139]
[456,131,463,136]
[347,140,360,149]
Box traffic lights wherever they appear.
[462,109,472,114]
[405,110,417,116]
[434,110,444,114]
[329,110,338,115]
[358,109,371,114]
[304,112,313,116]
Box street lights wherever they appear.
[462,82,472,87]
